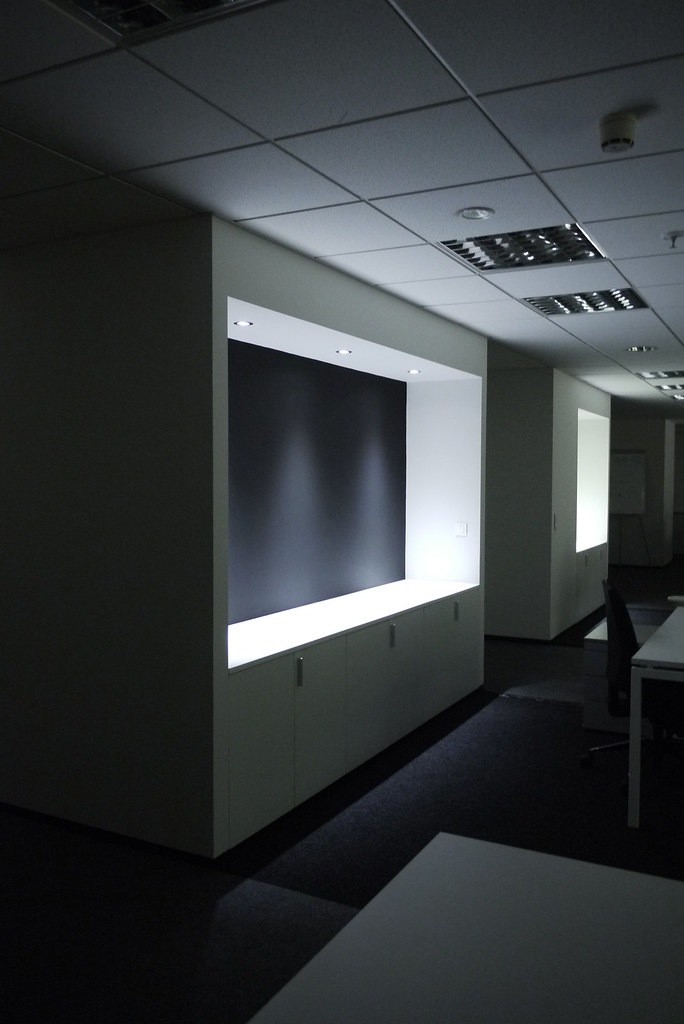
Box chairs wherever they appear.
[579,578,684,799]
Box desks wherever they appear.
[248,831,684,1024]
[627,605,684,829]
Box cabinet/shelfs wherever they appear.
[582,607,660,738]
[423,587,482,723]
[575,543,609,624]
[228,633,348,851]
[345,606,425,774]
